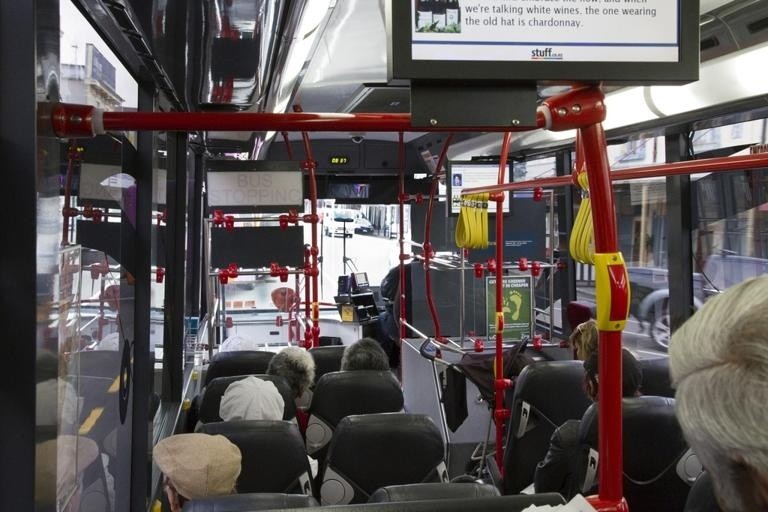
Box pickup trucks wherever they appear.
[625,250,768,349]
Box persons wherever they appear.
[534,349,643,499]
[36,348,67,382]
[35,436,99,512]
[153,433,242,512]
[266,346,316,433]
[371,264,400,339]
[570,319,599,361]
[340,338,390,371]
[667,274,768,512]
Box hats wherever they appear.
[583,349,643,389]
[34,435,98,501]
[152,433,243,500]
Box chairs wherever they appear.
[184,344,500,511]
[487,343,721,512]
[379,278,401,347]
[66,348,118,489]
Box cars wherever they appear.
[348,210,373,233]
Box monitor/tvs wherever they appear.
[384,0,699,86]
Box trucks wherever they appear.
[323,208,355,237]
